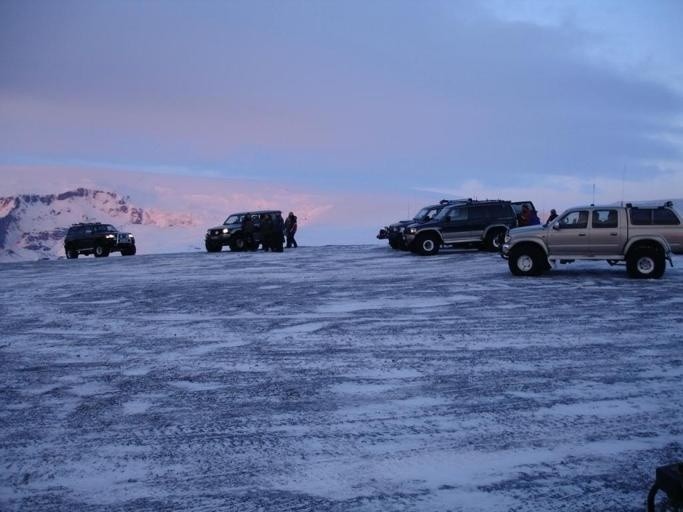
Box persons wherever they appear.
[517,204,531,226]
[571,219,576,224]
[578,213,588,224]
[546,209,559,263]
[605,213,616,224]
[241,212,298,252]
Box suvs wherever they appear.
[62,220,135,258]
[503,200,683,279]
[646,461,682,512]
[372,194,540,256]
[204,208,285,254]
